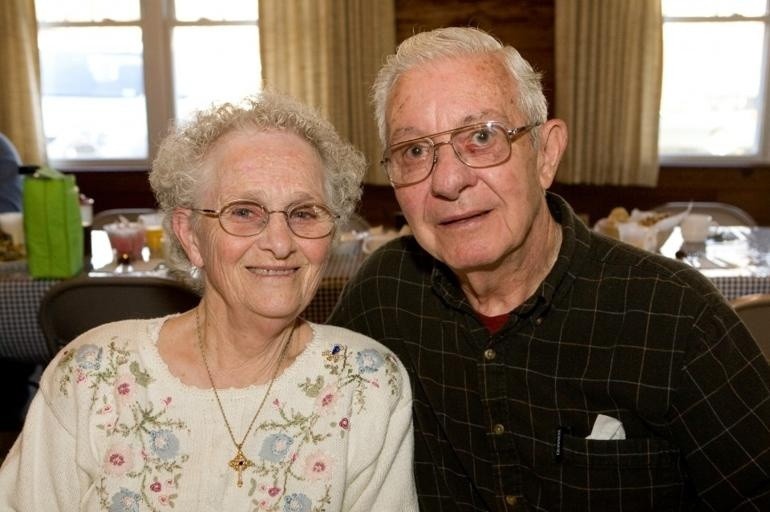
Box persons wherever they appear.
[0,128,30,228]
[0,83,422,511]
[320,23,770,511]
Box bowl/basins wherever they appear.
[598,222,675,251]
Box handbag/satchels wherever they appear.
[23,166,83,278]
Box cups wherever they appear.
[361,236,389,257]
[79,194,95,258]
[680,213,719,243]
[101,222,146,263]
[135,212,169,259]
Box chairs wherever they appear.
[37,276,202,360]
[92,209,154,230]
[729,294,770,361]
[343,213,370,232]
[654,203,756,226]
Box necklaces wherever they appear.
[187,307,301,492]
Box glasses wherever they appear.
[179,200,340,239]
[374,120,542,188]
